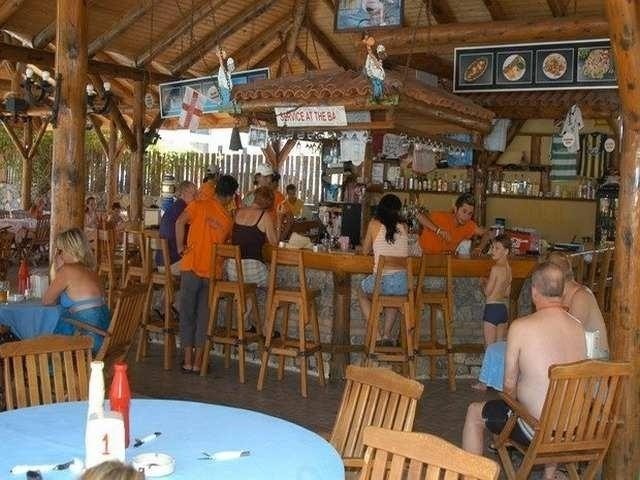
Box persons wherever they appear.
[156,181,198,277]
[358,193,409,347]
[544,249,611,408]
[226,186,286,339]
[29,197,44,219]
[43,228,111,394]
[458,264,591,480]
[106,202,126,247]
[174,175,238,375]
[262,172,285,213]
[202,174,215,185]
[253,172,265,186]
[472,234,513,393]
[82,196,101,255]
[413,194,492,255]
[278,184,305,220]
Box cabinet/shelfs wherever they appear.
[144,220,593,379]
[320,132,608,241]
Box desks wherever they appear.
[0,219,48,245]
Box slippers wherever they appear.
[262,331,282,340]
[245,325,258,334]
[181,360,192,373]
[193,366,211,376]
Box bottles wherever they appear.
[485,167,543,198]
[88,362,106,416]
[111,364,131,447]
[545,178,596,200]
[368,164,473,193]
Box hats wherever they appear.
[206,165,218,174]
[255,163,274,176]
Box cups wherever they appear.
[577,175,589,198]
[339,236,349,251]
[0,281,8,303]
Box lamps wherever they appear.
[87,82,114,130]
[143,123,161,153]
[21,68,63,128]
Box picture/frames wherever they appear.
[333,0,405,33]
[318,202,362,250]
[453,38,620,93]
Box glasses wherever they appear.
[58,248,62,254]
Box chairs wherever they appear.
[95,227,122,310]
[414,254,456,392]
[356,426,501,480]
[257,248,326,398]
[360,255,416,380]
[328,365,425,480]
[12,214,50,267]
[0,209,11,219]
[11,209,28,218]
[64,284,148,388]
[0,336,92,411]
[491,360,633,480]
[136,237,182,369]
[122,230,147,356]
[0,231,15,272]
[200,243,264,383]
